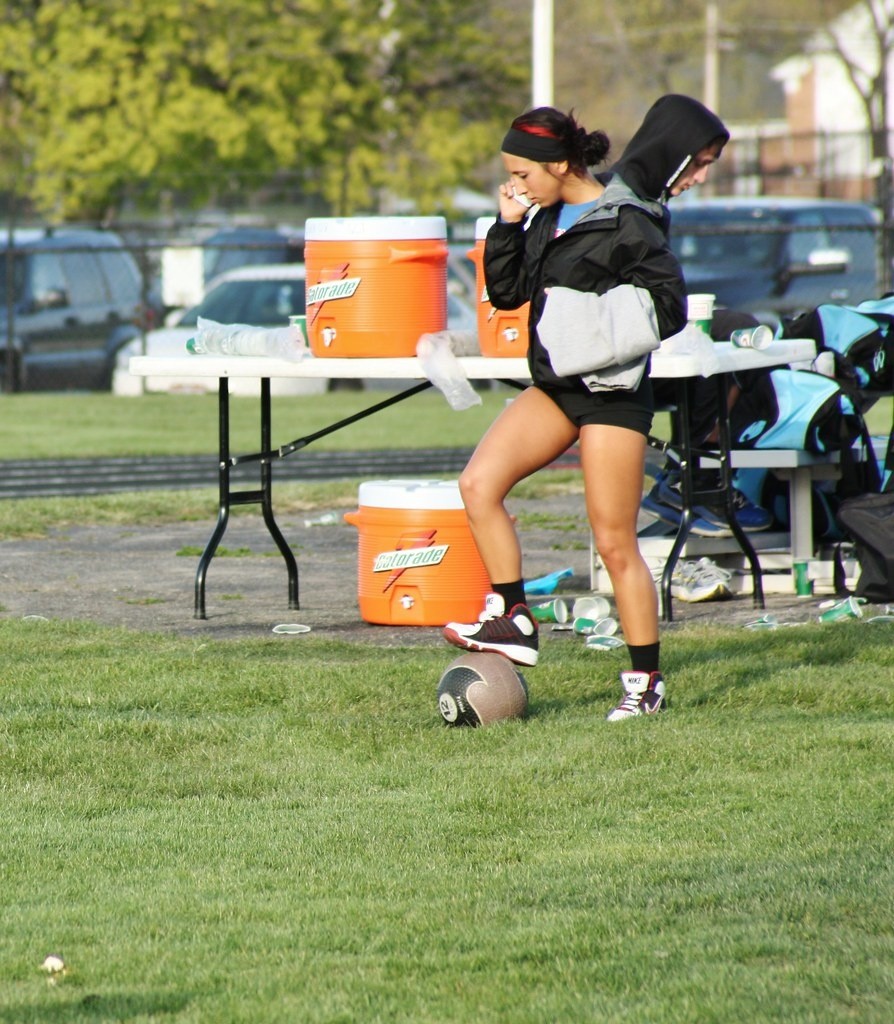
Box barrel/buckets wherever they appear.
[343,478,498,627]
[465,215,566,355]
[300,214,449,358]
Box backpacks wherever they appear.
[723,284,894,493]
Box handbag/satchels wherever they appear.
[833,490,894,600]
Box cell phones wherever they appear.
[511,187,532,207]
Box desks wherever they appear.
[126,337,819,620]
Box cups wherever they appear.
[288,314,308,347]
[740,613,802,631]
[791,561,816,599]
[730,325,774,352]
[571,596,625,652]
[526,598,568,624]
[186,335,203,354]
[867,605,894,625]
[271,624,312,635]
[686,293,718,341]
[817,595,867,626]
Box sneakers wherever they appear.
[443,591,542,669]
[605,672,674,722]
[660,465,777,532]
[640,469,740,538]
[669,556,735,603]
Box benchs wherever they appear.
[698,442,892,583]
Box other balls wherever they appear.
[436,651,530,727]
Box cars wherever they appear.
[112,263,493,398]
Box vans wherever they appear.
[1,224,308,392]
[668,194,879,323]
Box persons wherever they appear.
[442,107,688,723]
[592,95,779,538]
